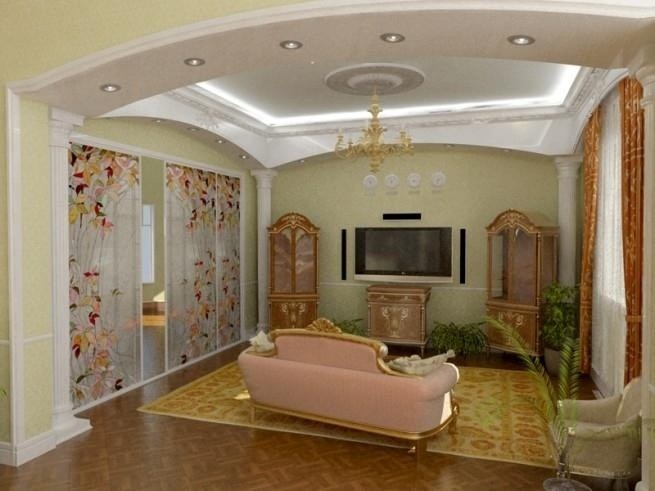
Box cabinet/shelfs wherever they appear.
[267,212,321,334]
[483,206,560,364]
[365,284,432,355]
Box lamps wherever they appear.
[332,84,416,176]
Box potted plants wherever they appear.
[540,280,580,377]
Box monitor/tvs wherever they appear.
[347,224,461,285]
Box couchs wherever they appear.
[239,318,462,458]
[548,376,643,489]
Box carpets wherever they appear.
[137,355,561,469]
